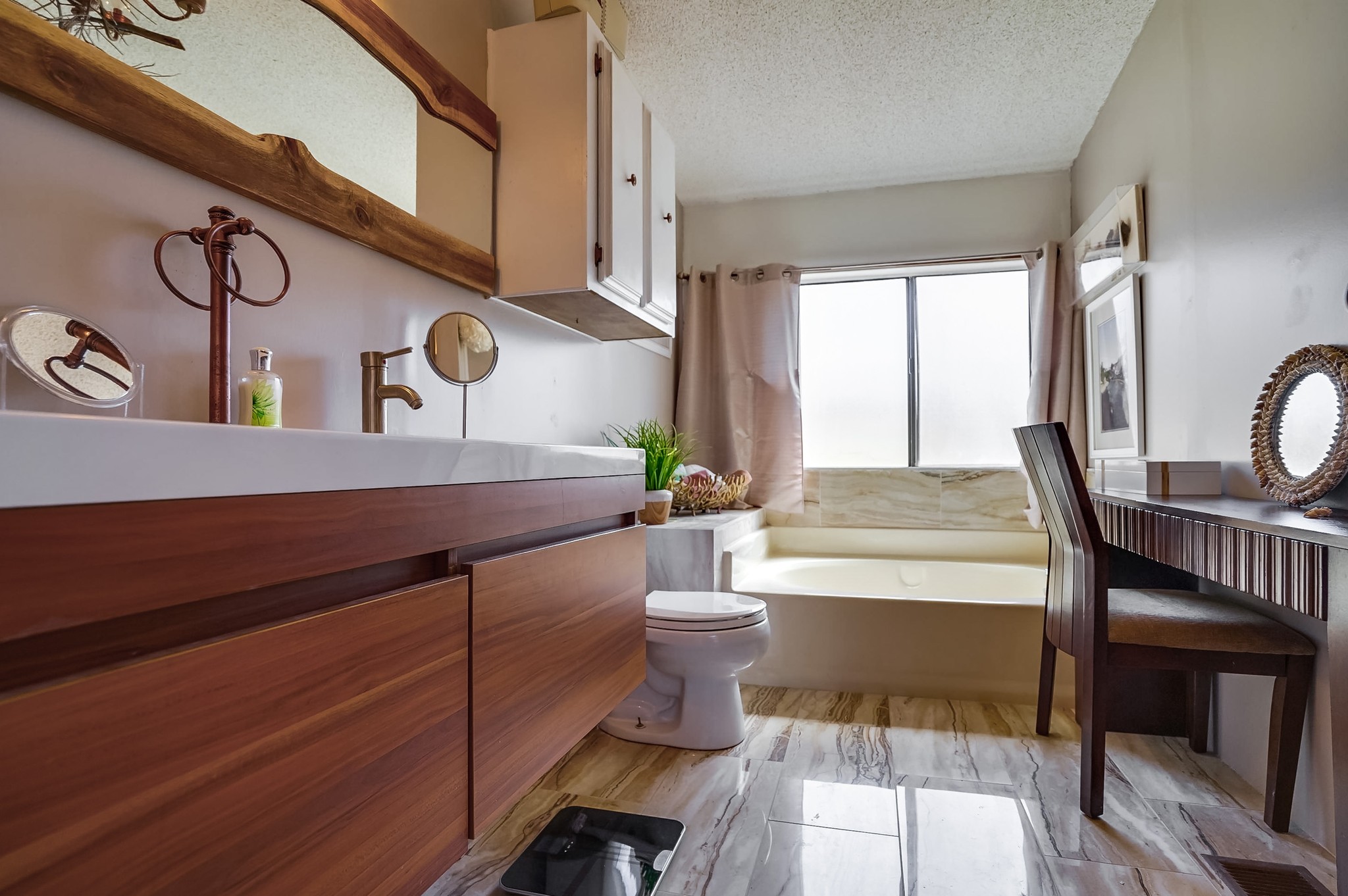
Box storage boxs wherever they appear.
[532,0,631,62]
[1093,457,1224,497]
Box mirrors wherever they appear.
[1248,343,1348,508]
[0,0,502,300]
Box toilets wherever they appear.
[599,589,771,751]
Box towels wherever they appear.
[680,469,717,499]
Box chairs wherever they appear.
[1008,420,1318,837]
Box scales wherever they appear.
[501,807,685,896]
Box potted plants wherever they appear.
[599,415,714,528]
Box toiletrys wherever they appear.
[235,349,284,431]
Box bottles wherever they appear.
[237,346,283,427]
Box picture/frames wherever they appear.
[1082,271,1148,460]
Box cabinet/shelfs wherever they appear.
[484,10,680,343]
[464,522,650,844]
[0,569,469,896]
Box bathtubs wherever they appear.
[723,524,1074,701]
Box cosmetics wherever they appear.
[238,347,283,429]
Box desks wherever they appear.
[1077,480,1348,896]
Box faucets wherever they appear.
[359,347,422,435]
